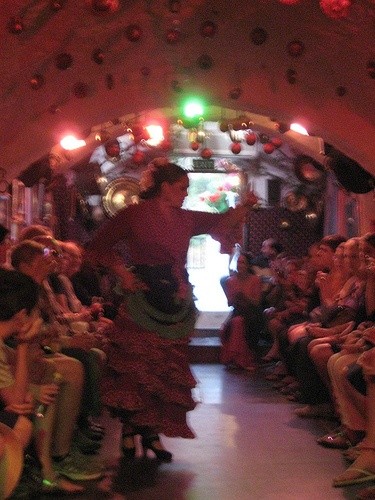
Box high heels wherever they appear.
[121,432,135,461]
[142,437,172,459]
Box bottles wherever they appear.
[34,373,62,418]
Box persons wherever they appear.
[99,163,257,462]
[219,234,375,500]
[0,223,119,500]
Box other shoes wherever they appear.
[280,384,298,393]
[294,405,330,416]
[81,448,96,455]
[344,442,361,455]
[89,422,103,428]
[289,391,303,402]
[264,366,286,380]
[272,376,291,388]
[321,430,358,448]
[256,354,279,365]
[346,440,375,465]
[41,477,86,494]
[89,426,102,432]
[317,427,341,443]
[89,433,103,441]
[77,439,100,448]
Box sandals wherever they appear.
[332,468,375,488]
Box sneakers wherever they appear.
[69,452,106,469]
[53,457,104,481]
